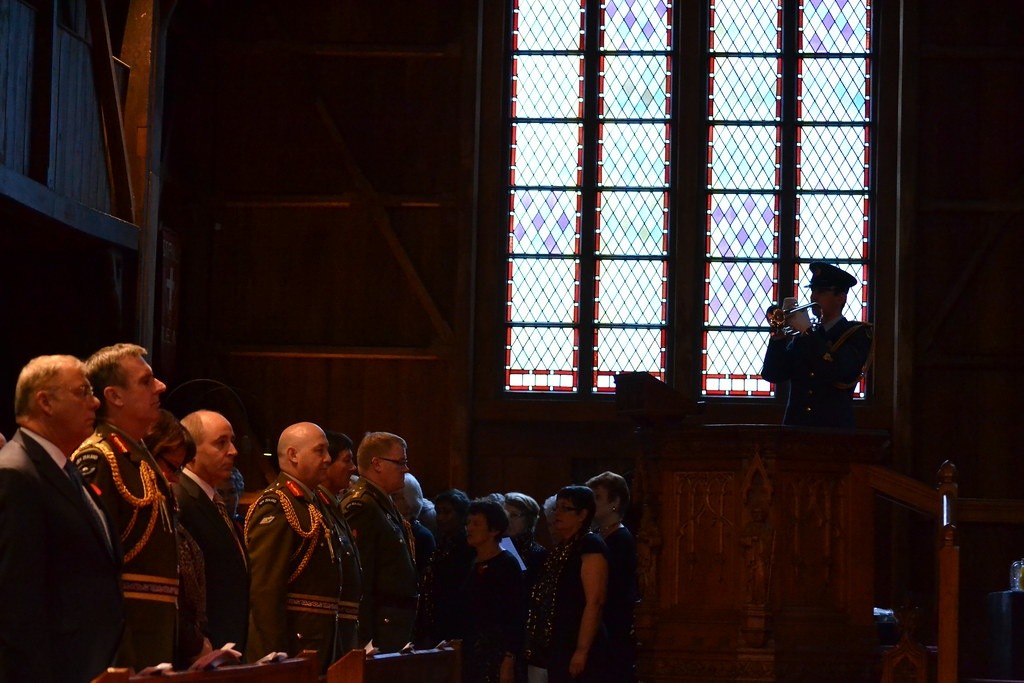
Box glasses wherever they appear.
[156,455,182,480]
[372,455,408,468]
[554,504,577,518]
[510,510,524,519]
[51,385,96,406]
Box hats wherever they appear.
[805,258,857,294]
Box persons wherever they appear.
[421,491,525,683]
[147,408,251,667]
[67,342,179,674]
[586,472,637,683]
[0,354,125,683]
[525,486,612,683]
[392,472,435,574]
[242,421,341,674]
[314,430,363,653]
[761,262,870,428]
[502,492,546,582]
[339,432,420,656]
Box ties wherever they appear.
[64,458,111,553]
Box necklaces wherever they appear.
[599,522,619,533]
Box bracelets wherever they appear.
[504,654,516,659]
